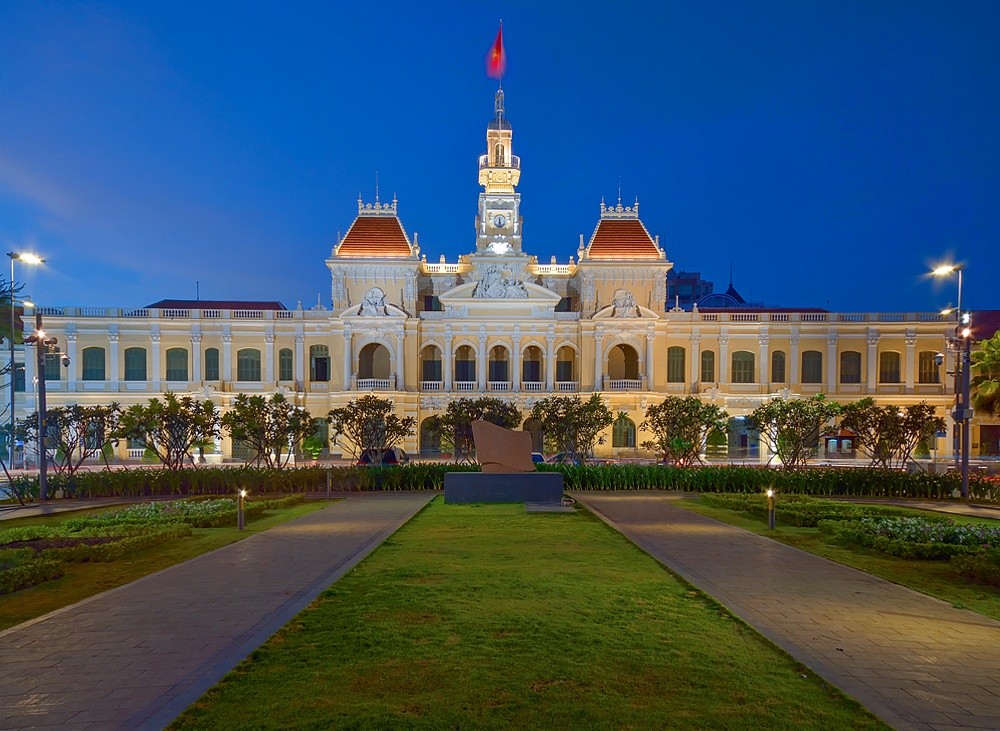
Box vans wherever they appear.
[358,447,410,465]
[547,451,593,463]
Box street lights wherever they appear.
[932,264,974,502]
[6,253,43,469]
[35,313,71,500]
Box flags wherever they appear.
[487,23,505,81]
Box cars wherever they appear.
[530,451,546,463]
